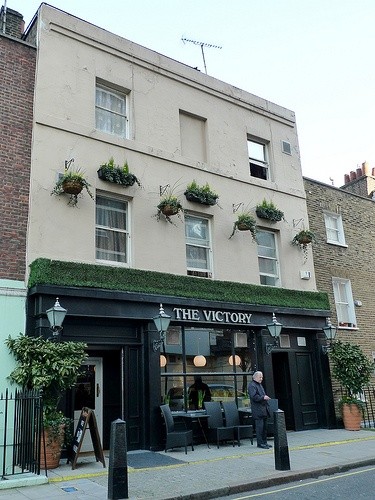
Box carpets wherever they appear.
[105,452,189,469]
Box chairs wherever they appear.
[221,402,253,447]
[160,404,194,455]
[204,402,236,449]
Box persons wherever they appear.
[248,371,273,449]
[187,376,211,410]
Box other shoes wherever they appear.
[257,443,272,449]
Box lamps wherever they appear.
[229,354,241,366]
[194,346,206,367]
[321,317,336,354]
[160,355,166,368]
[46,298,67,340]
[153,304,170,349]
[267,313,282,355]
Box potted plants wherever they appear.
[5,332,88,469]
[292,229,320,266]
[155,177,185,227]
[183,179,223,210]
[330,340,374,431]
[52,167,95,207]
[97,161,142,188]
[228,202,259,245]
[256,197,284,222]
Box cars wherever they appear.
[167,384,250,412]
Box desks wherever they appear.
[238,409,256,445]
[171,413,211,450]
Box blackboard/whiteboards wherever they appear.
[67,407,104,463]
